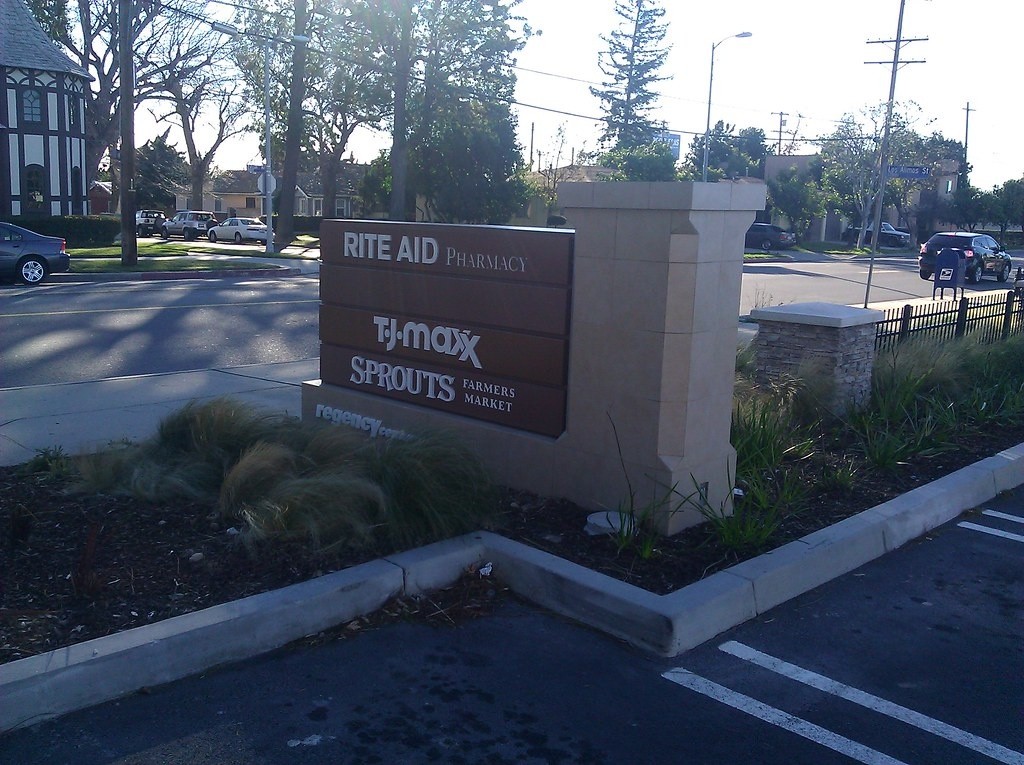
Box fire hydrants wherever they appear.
[1014,266,1024,281]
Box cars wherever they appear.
[847,221,910,247]
[745,222,797,251]
[0,222,71,286]
[207,217,275,246]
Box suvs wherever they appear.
[918,231,1012,285]
[161,210,219,242]
[136,210,167,238]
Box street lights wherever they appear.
[210,20,310,254]
[702,31,752,183]
